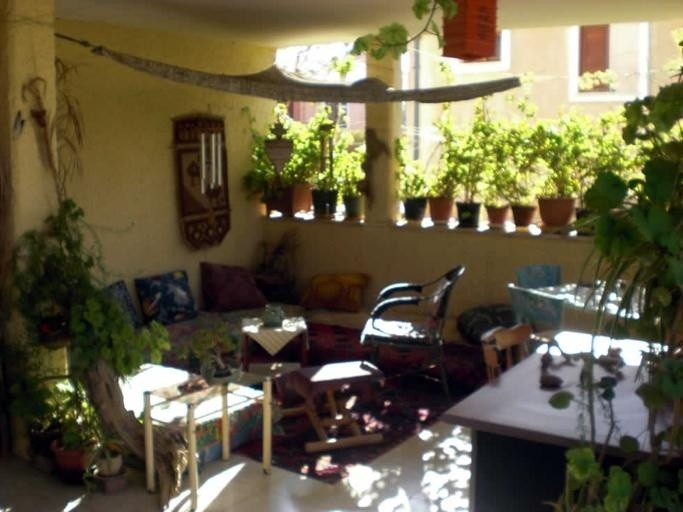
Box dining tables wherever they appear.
[348,0,497,61]
[175,311,244,384]
[392,62,627,236]
[7,374,129,498]
[241,102,365,221]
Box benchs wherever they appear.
[150,302,458,371]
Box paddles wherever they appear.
[227,345,487,485]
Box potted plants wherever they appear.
[7,374,129,498]
[348,0,497,61]
[175,311,244,384]
[392,62,627,236]
[241,102,365,221]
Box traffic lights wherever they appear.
[95,260,368,331]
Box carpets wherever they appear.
[227,345,487,485]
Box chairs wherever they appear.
[479,321,534,383]
[359,264,466,396]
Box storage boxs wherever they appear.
[150,302,458,371]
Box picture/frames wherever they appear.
[505,278,669,343]
[141,367,272,509]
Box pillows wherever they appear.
[95,260,368,331]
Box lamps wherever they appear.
[479,321,534,383]
[359,264,466,396]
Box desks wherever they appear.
[141,367,272,509]
[505,278,669,343]
[440,322,683,512]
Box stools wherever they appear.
[281,358,383,453]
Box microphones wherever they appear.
[281,358,383,453]
